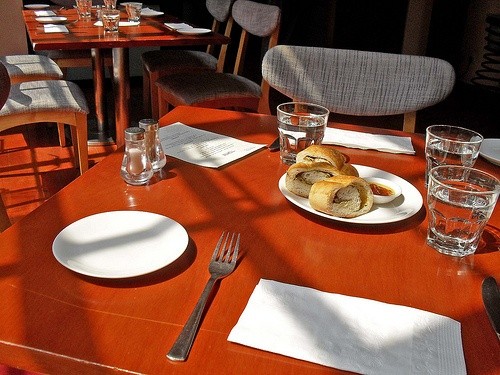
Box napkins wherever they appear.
[319,127,416,155]
[93,20,139,26]
[165,23,193,30]
[43,24,70,33]
[226,278,467,375]
[34,10,57,16]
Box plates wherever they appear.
[139,11,164,17]
[51,208,189,279]
[177,27,211,36]
[24,4,49,9]
[35,17,68,24]
[278,164,424,225]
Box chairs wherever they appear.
[0,54,89,176]
[140,0,455,132]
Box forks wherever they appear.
[165,231,241,362]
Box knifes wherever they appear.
[269,137,280,151]
[481,275,500,341]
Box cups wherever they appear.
[425,166,500,257]
[76,0,92,20]
[100,9,120,34]
[104,0,117,10]
[276,102,330,166]
[424,124,483,189]
[126,3,142,24]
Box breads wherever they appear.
[286,145,373,218]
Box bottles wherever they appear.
[139,118,167,173]
[120,127,154,186]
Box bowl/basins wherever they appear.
[365,178,402,204]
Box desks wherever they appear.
[0,104,500,375]
[22,6,231,150]
